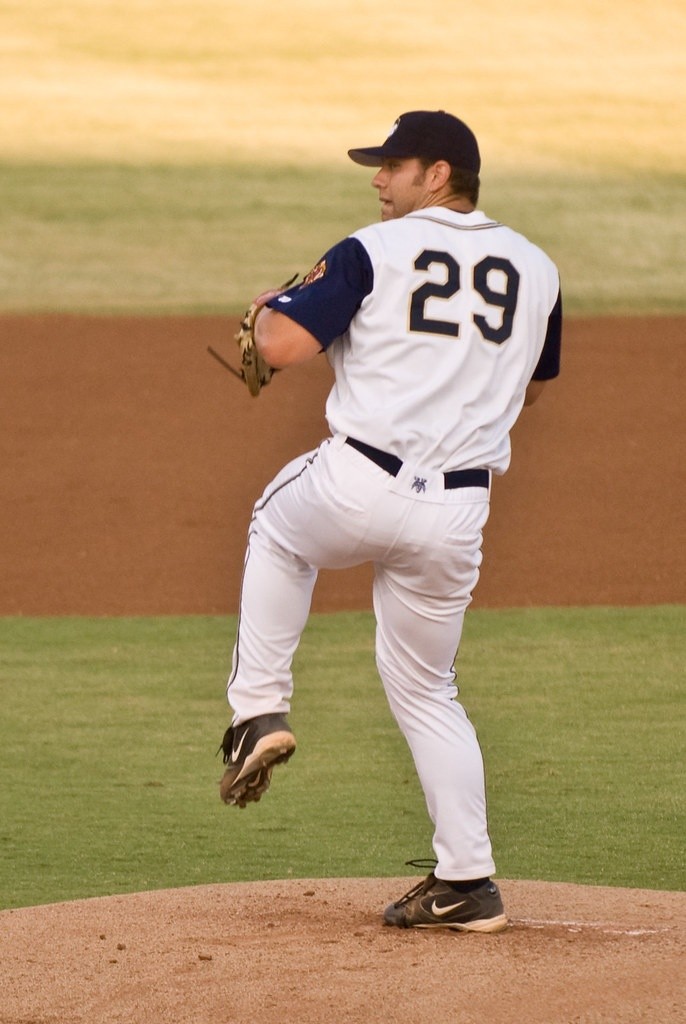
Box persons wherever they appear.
[220,109,561,933]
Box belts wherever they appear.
[346,437,489,490]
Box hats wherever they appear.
[348,110,481,170]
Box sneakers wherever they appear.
[220,713,297,807]
[382,871,508,933]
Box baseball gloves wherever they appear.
[208,272,299,397]
